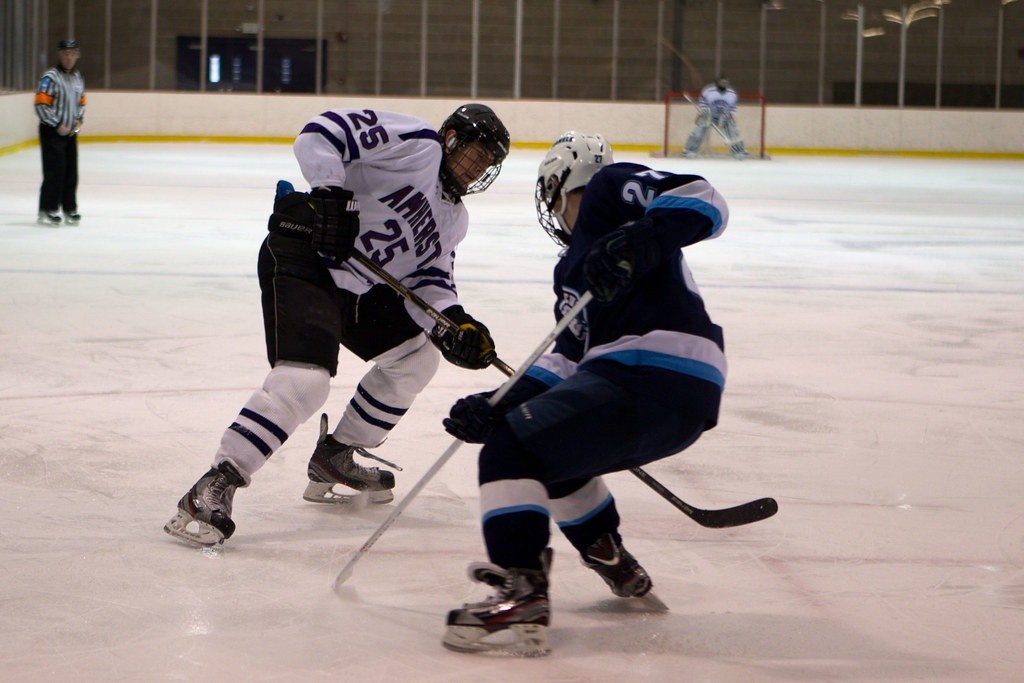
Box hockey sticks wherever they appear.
[350,247,779,532]
[333,291,594,604]
[683,93,745,146]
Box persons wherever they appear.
[34,39,87,227]
[442,132,729,656]
[681,76,749,156]
[166,103,510,550]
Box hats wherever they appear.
[57,40,80,48]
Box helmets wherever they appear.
[718,79,729,92]
[436,104,511,198]
[534,130,614,248]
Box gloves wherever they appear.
[694,108,711,127]
[444,388,532,443]
[582,219,659,302]
[718,114,728,128]
[429,305,497,370]
[310,186,361,267]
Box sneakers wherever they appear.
[303,413,404,504]
[163,457,250,555]
[63,208,81,225]
[445,547,555,656]
[37,207,62,227]
[580,527,669,614]
